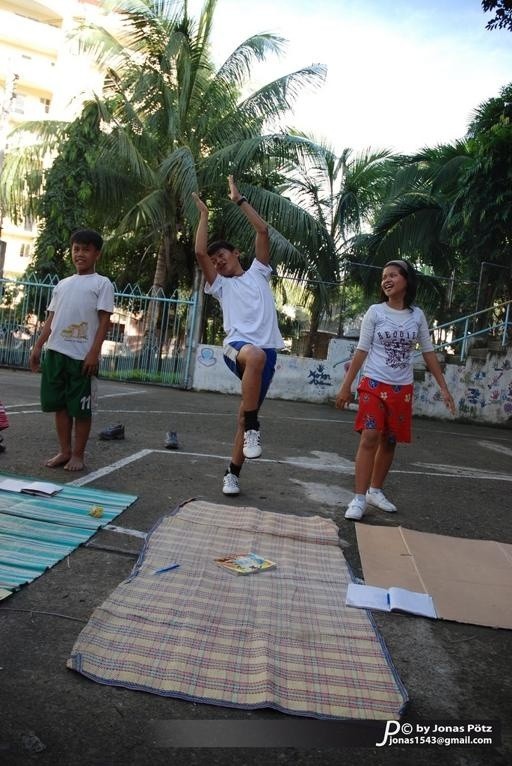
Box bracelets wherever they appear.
[236,195,247,206]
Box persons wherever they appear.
[29,230,115,472]
[191,174,288,498]
[333,258,456,524]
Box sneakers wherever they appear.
[100,424,124,440]
[366,490,398,512]
[242,430,262,458]
[165,430,178,449]
[222,474,240,496]
[344,496,367,520]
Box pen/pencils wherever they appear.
[150,563,179,575]
[387,593,390,609]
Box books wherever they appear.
[343,582,439,621]
[212,551,277,576]
[0,477,65,499]
